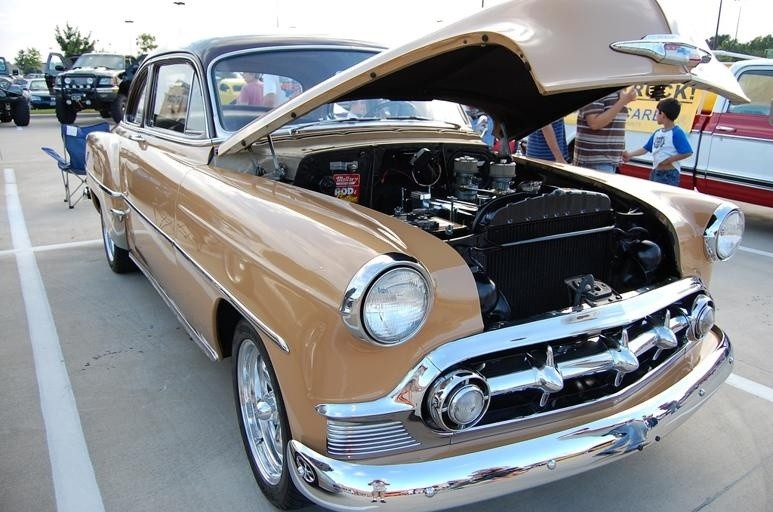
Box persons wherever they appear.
[573,85,637,175]
[526,116,570,164]
[259,72,304,109]
[462,100,527,156]
[620,97,693,188]
[233,72,262,107]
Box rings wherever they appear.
[630,93,633,97]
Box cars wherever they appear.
[0,53,147,126]
[79,0,750,511]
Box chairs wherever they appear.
[39,121,109,210]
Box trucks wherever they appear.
[518,47,772,215]
